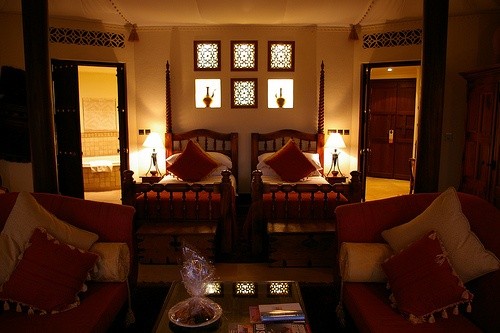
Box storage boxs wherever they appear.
[137,221,217,265]
[266,220,336,268]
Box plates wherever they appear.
[168,301,223,328]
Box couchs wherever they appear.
[0,192,136,333]
[334,192,500,333]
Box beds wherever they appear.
[250,61,363,259]
[121,59,238,255]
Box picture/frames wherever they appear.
[204,87,212,107]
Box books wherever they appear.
[249,302,306,323]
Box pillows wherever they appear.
[255,138,324,182]
[381,185,500,284]
[340,241,393,282]
[87,242,132,283]
[0,192,100,285]
[0,226,101,322]
[379,230,474,325]
[165,139,233,181]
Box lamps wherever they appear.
[143,130,163,177]
[325,132,347,176]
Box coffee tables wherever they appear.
[151,279,311,333]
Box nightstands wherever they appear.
[325,176,348,186]
[139,176,162,184]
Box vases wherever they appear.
[277,89,285,108]
